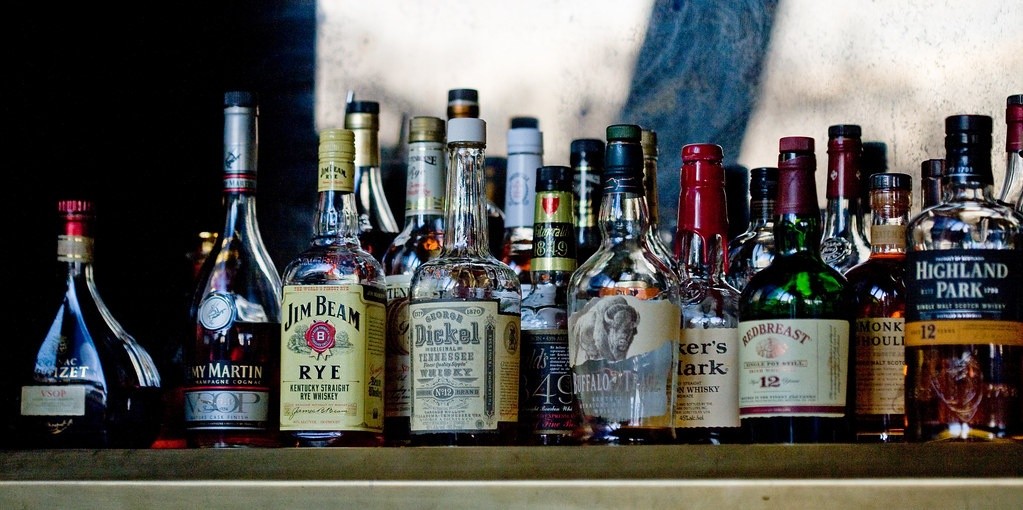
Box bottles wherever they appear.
[181,88,1023,447]
[34,200,165,449]
